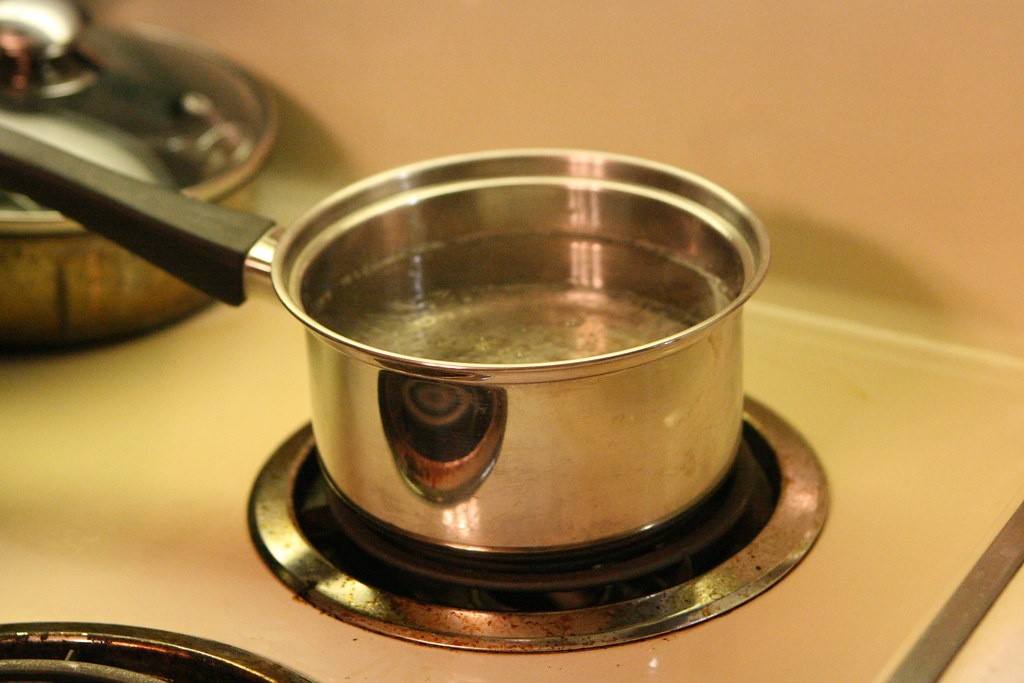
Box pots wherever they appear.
[0,0,283,352]
[0,124,771,552]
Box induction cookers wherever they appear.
[0,175,1024,683]
